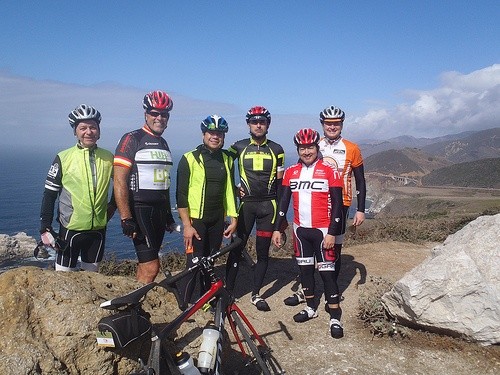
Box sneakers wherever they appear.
[330,318,344,338]
[209,292,235,311]
[249,295,270,312]
[284,290,306,305]
[293,306,318,323]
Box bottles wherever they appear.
[174,350,202,375]
[197,322,219,374]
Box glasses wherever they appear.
[148,111,169,117]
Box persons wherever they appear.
[39,102,118,272]
[223,104,289,312]
[270,126,344,339]
[112,88,178,286]
[176,114,240,312]
[283,104,366,306]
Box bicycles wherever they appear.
[94,234,284,375]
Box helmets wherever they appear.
[143,91,173,111]
[69,105,101,127]
[320,106,345,122]
[201,115,228,133]
[245,106,271,123]
[293,128,319,145]
